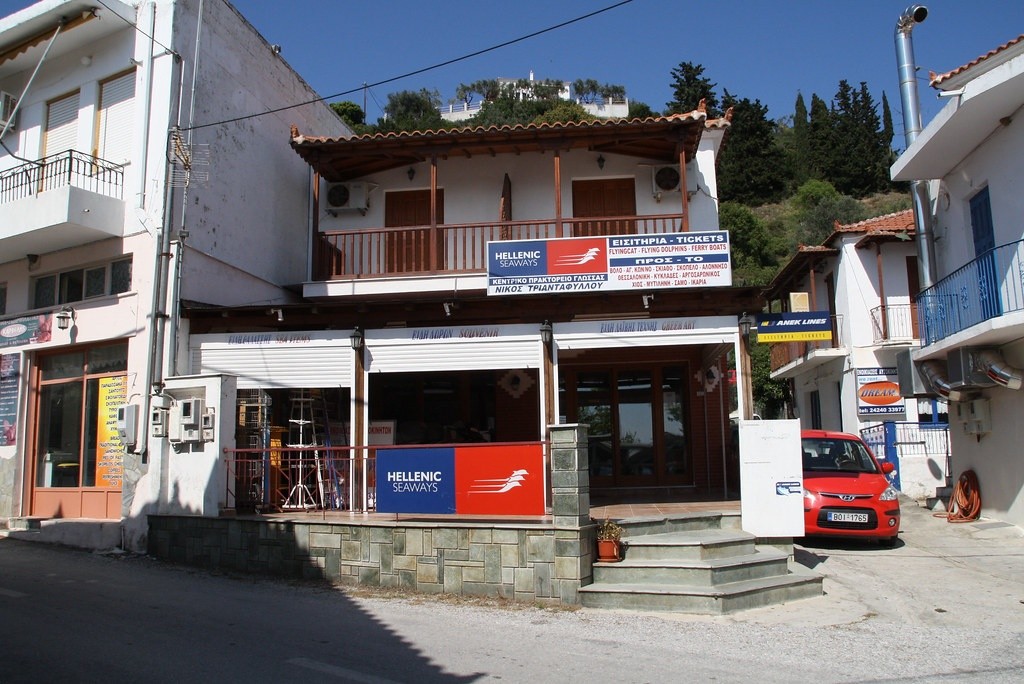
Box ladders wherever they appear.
[308,392,342,510]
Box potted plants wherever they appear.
[597,515,627,562]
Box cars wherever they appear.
[800,430,901,547]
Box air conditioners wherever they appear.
[651,162,701,203]
[323,181,380,218]
[0,90,18,134]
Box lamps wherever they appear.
[895,222,916,241]
[444,302,453,316]
[27,254,41,272]
[509,376,521,391]
[407,167,416,181]
[597,155,606,170]
[706,369,716,384]
[271,308,284,322]
[642,294,654,309]
[56,304,78,330]
[81,55,92,65]
